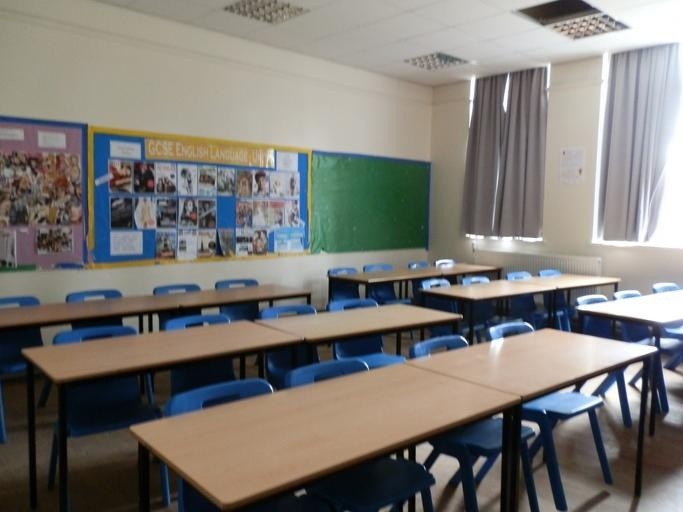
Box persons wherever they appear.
[255,171,267,194]
[253,230,269,252]
[137,162,153,190]
[181,196,198,224]
[201,201,216,228]
[287,200,300,226]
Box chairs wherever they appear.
[1,257,682,512]
